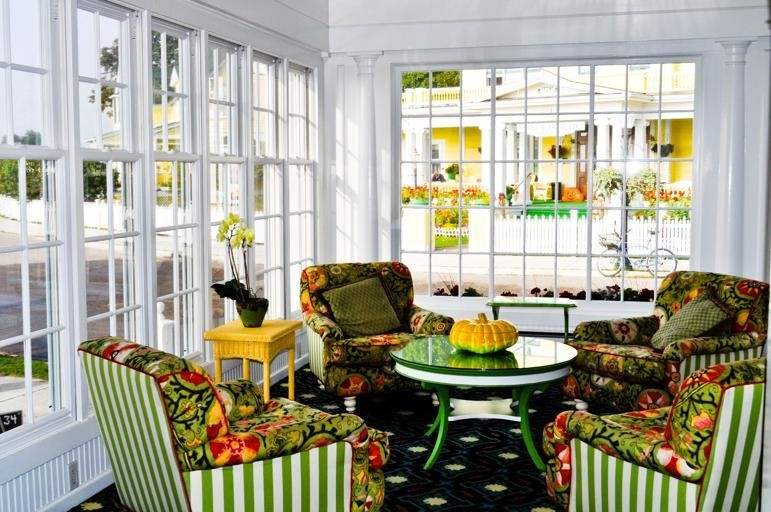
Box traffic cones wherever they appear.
[443,352,518,369]
[448,313,520,354]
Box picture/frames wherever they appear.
[486,296,577,340]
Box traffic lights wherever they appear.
[649,294,729,350]
[319,277,401,339]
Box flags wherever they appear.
[233,298,270,328]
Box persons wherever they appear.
[432,171,445,182]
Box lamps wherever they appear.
[297,262,455,415]
[79,335,394,511]
[563,266,770,413]
[538,356,770,511]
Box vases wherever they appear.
[207,212,264,309]
